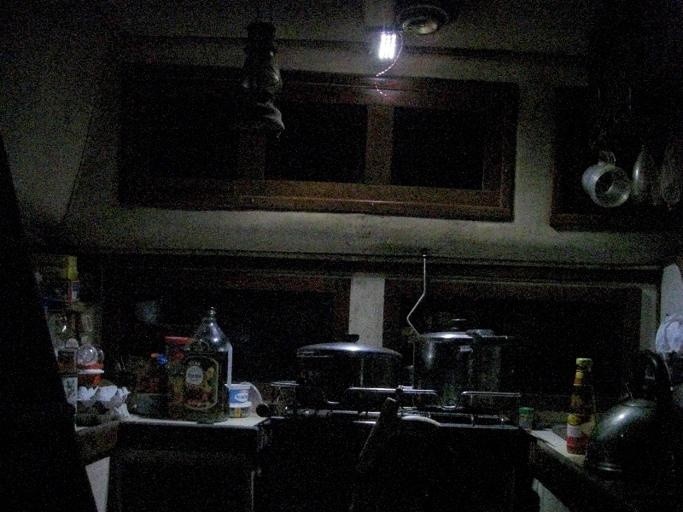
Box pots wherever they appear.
[295,332,405,409]
[409,318,519,411]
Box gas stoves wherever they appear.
[268,395,528,435]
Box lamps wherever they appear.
[374,32,399,62]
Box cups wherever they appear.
[225,383,250,406]
[583,149,632,208]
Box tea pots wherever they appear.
[588,351,682,480]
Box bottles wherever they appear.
[57,348,79,414]
[566,357,596,453]
